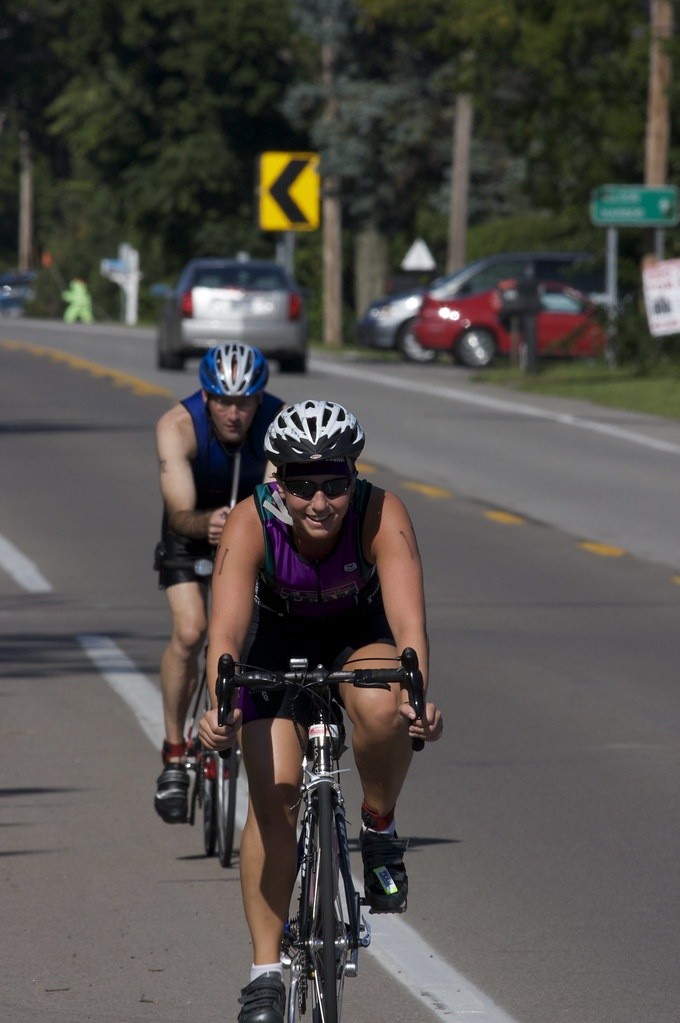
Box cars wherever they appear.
[418,280,633,370]
[0,271,41,310]
[149,256,310,376]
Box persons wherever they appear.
[499,275,539,375]
[154,342,288,825]
[200,399,444,1022]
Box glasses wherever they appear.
[278,476,353,499]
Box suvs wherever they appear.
[357,250,645,364]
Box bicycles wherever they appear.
[168,575,245,870]
[215,642,437,1023]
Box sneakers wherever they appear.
[359,823,408,912]
[153,764,189,824]
[237,972,286,1023]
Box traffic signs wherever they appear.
[590,184,679,227]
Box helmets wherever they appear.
[198,340,268,398]
[263,400,367,467]
